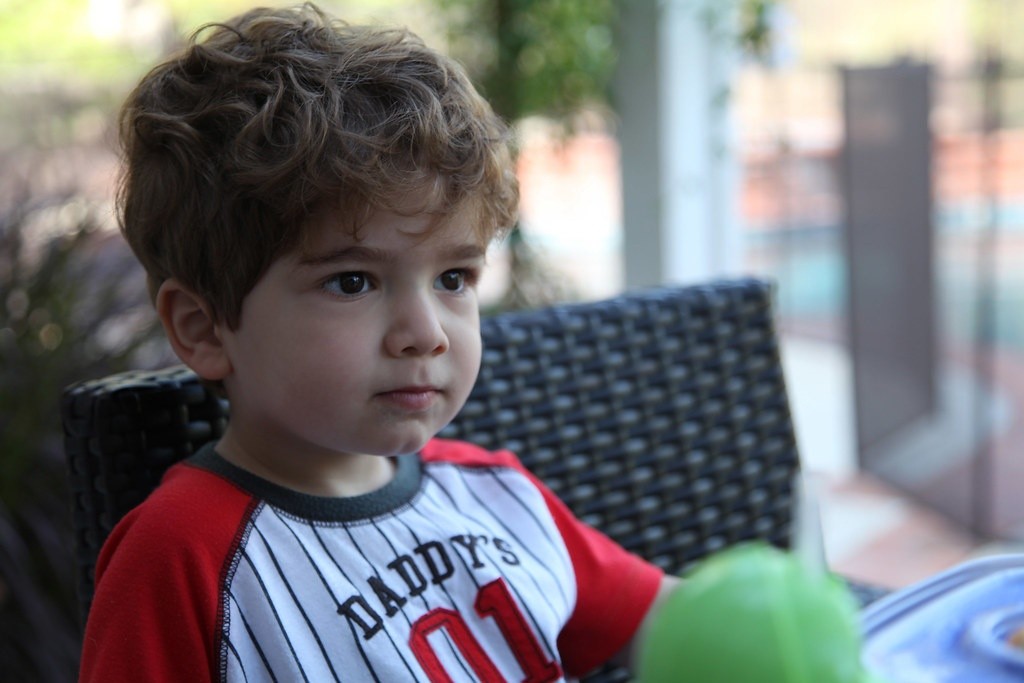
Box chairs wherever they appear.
[60,280,1023,683]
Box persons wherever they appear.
[78,0,691,683]
